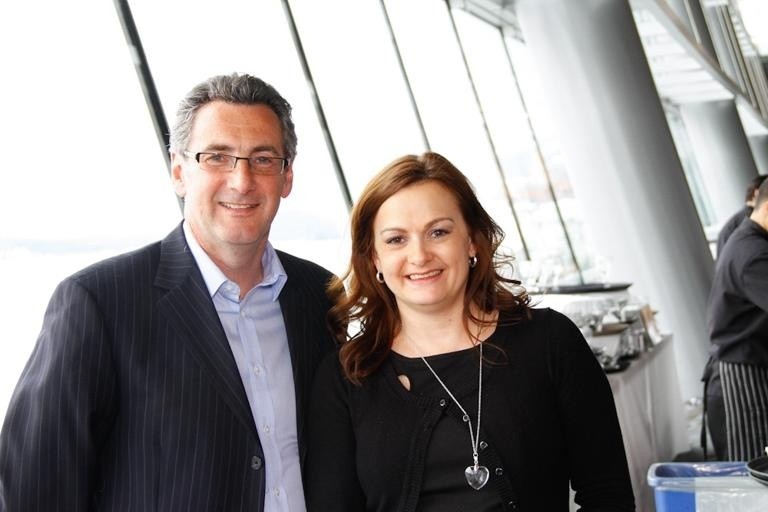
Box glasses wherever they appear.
[184,150,288,177]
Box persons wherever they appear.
[704,176,768,462]
[307,151,635,512]
[716,175,765,257]
[0,72,350,512]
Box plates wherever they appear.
[747,456,768,489]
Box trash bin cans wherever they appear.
[646,461,750,512]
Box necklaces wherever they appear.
[400,313,491,490]
